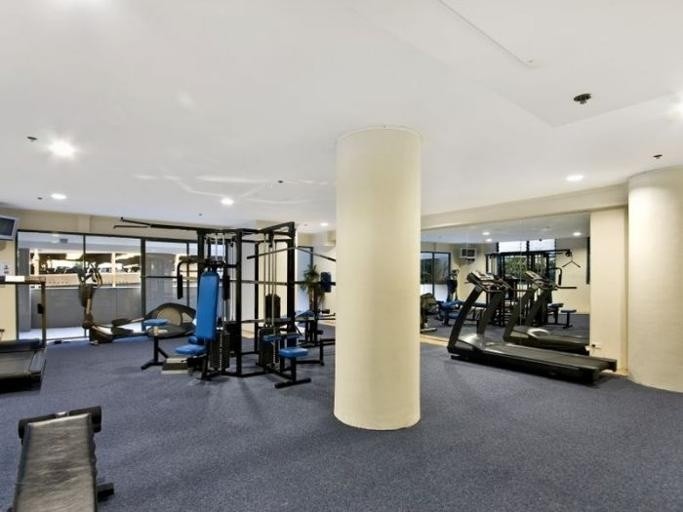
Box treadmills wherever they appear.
[0,276,47,394]
[447,271,617,385]
[502,270,590,356]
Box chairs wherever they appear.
[175,270,220,381]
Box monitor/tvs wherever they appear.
[460,248,477,258]
[0,214,20,240]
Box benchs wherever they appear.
[6,406,113,511]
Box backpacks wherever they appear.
[421,293,439,313]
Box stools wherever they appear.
[141,318,169,370]
